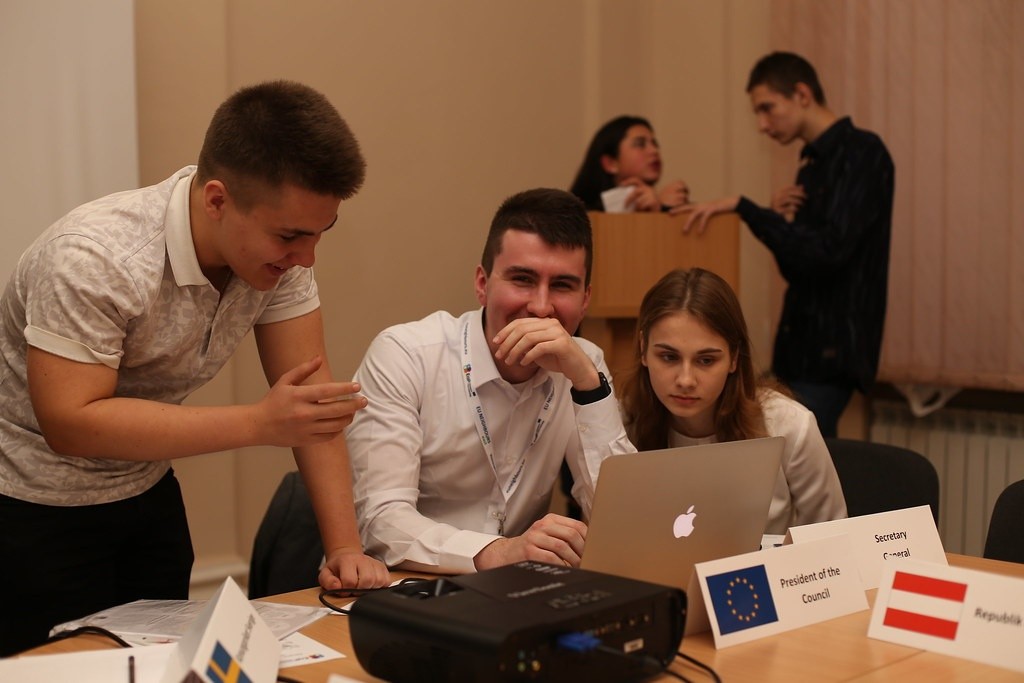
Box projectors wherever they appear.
[347,558,688,683]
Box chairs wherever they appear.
[983,479,1024,564]
[830,439,939,528]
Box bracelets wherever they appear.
[570,372,611,405]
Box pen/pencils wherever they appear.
[128,656,136,683]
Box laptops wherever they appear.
[580,436,785,636]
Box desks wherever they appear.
[0,531,1024,683]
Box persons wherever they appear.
[620,267,848,536]
[670,50,895,434]
[343,187,639,575]
[0,79,390,659]
[568,116,688,212]
